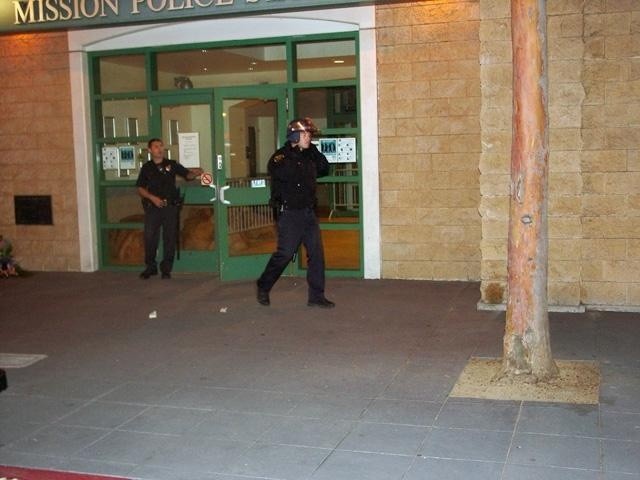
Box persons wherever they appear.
[135,138,205,281]
[256,117,338,309]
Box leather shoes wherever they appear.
[256,285,270,305]
[307,297,335,309]
[161,272,170,279]
[140,266,157,279]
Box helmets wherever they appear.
[287,118,320,142]
